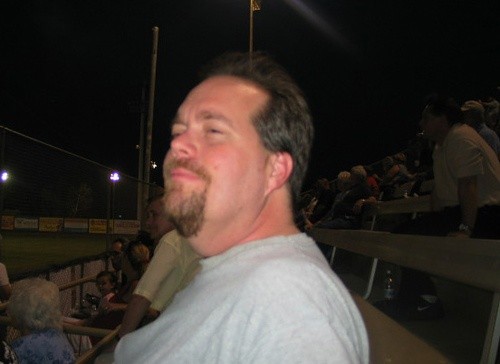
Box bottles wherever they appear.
[384,270,395,301]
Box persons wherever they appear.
[295,87,500,320]
[0,193,200,364]
[115,51,368,364]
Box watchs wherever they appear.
[457,223,473,234]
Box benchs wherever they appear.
[310,182,500,364]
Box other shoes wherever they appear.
[404,301,441,320]
